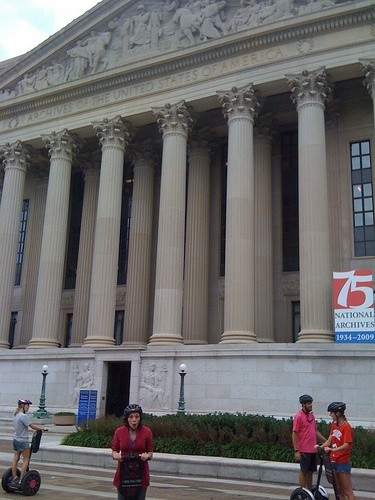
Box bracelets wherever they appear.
[293,450,298,453]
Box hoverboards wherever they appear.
[113,456,152,500]
[290,446,331,500]
[2,429,48,496]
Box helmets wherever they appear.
[328,402,346,412]
[299,395,312,404]
[18,397,32,406]
[124,403,143,418]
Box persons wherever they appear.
[291,394,327,491]
[16,0,332,95]
[314,401,354,500]
[11,399,43,484]
[112,404,153,500]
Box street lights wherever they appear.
[37,364,49,415]
[176,364,188,416]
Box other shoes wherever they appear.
[19,478,22,484]
[11,475,18,481]
